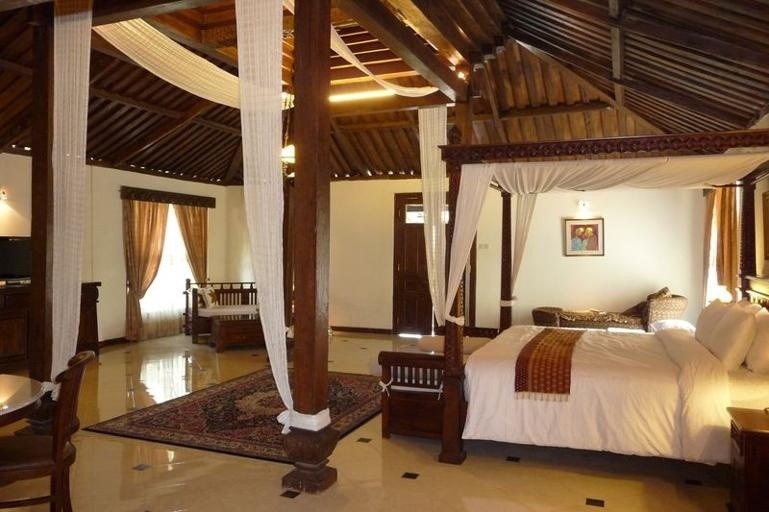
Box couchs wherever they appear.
[182,278,295,344]
[532,290,689,333]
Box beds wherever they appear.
[435,126,769,466]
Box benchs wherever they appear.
[377,325,499,441]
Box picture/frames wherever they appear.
[565,218,604,256]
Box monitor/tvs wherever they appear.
[0,150,33,243]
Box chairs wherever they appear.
[0,350,97,512]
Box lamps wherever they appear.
[277,84,297,187]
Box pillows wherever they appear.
[199,286,219,309]
[418,334,492,354]
[641,286,672,328]
[695,296,769,373]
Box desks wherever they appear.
[0,373,46,426]
[0,281,102,371]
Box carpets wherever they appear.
[80,362,382,465]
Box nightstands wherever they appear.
[724,406,769,512]
